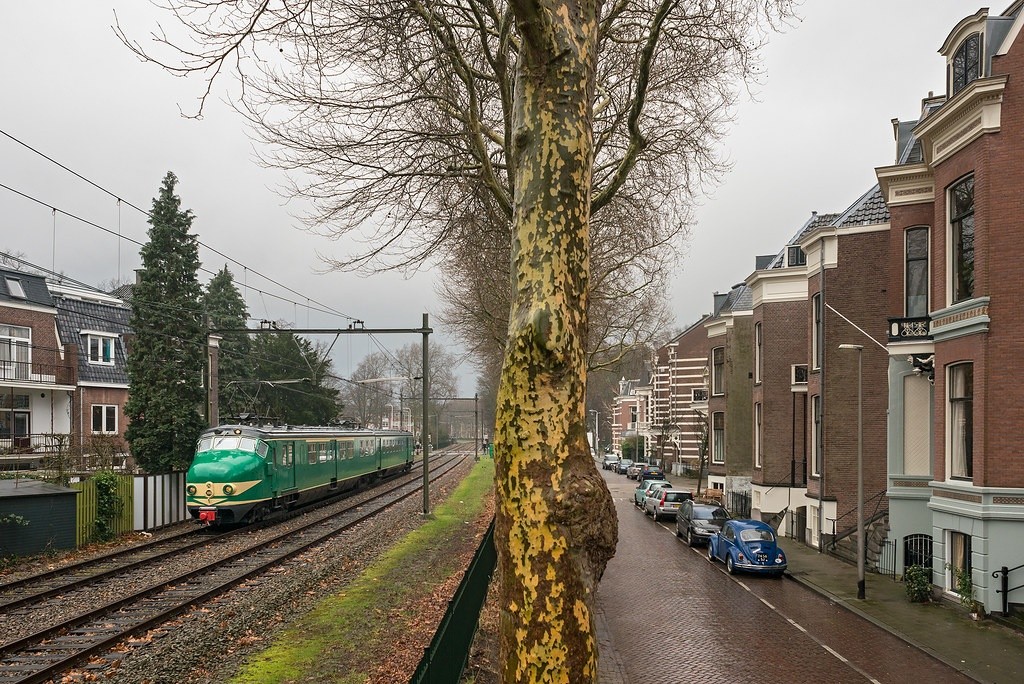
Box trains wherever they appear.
[185,420,417,530]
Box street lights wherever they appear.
[589,409,599,457]
[403,408,412,432]
[839,344,869,598]
[385,404,394,429]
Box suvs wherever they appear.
[603,455,620,470]
[676,499,732,547]
[618,459,634,474]
[626,463,650,480]
[637,465,664,483]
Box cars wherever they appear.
[634,480,673,507]
[707,519,788,575]
[604,444,612,452]
[642,488,694,521]
